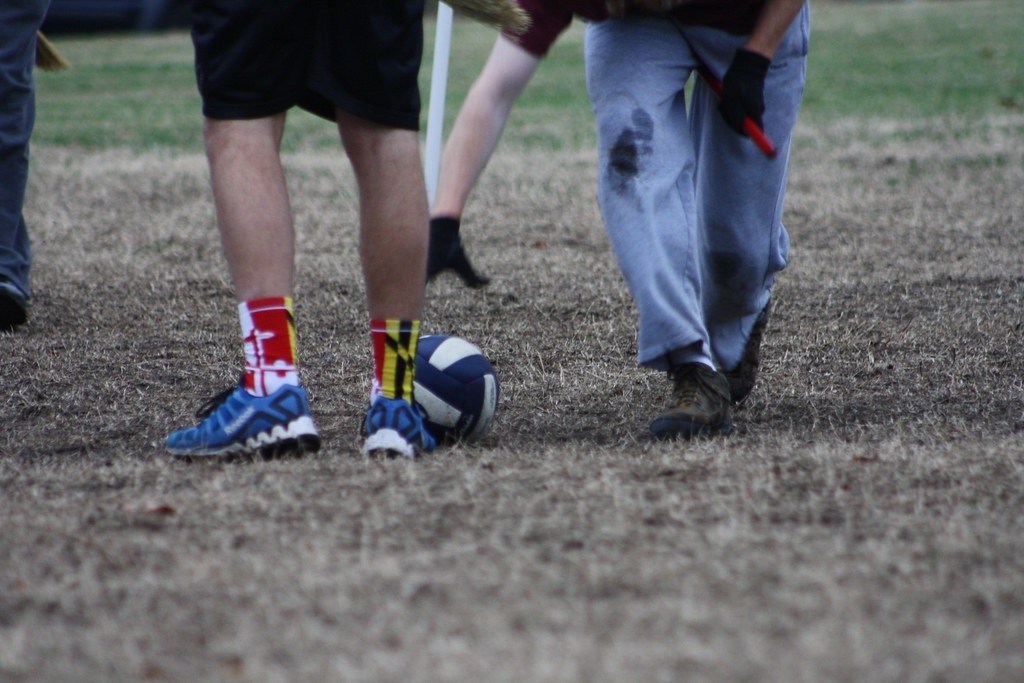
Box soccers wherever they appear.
[367,332,498,446]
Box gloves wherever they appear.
[426,214,491,291]
[715,46,773,139]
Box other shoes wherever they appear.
[0,286,27,333]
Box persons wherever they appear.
[425,1,811,443]
[0,0,50,335]
[160,0,436,459]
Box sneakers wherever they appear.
[166,371,321,463]
[712,298,771,407]
[361,395,435,460]
[649,362,732,437]
